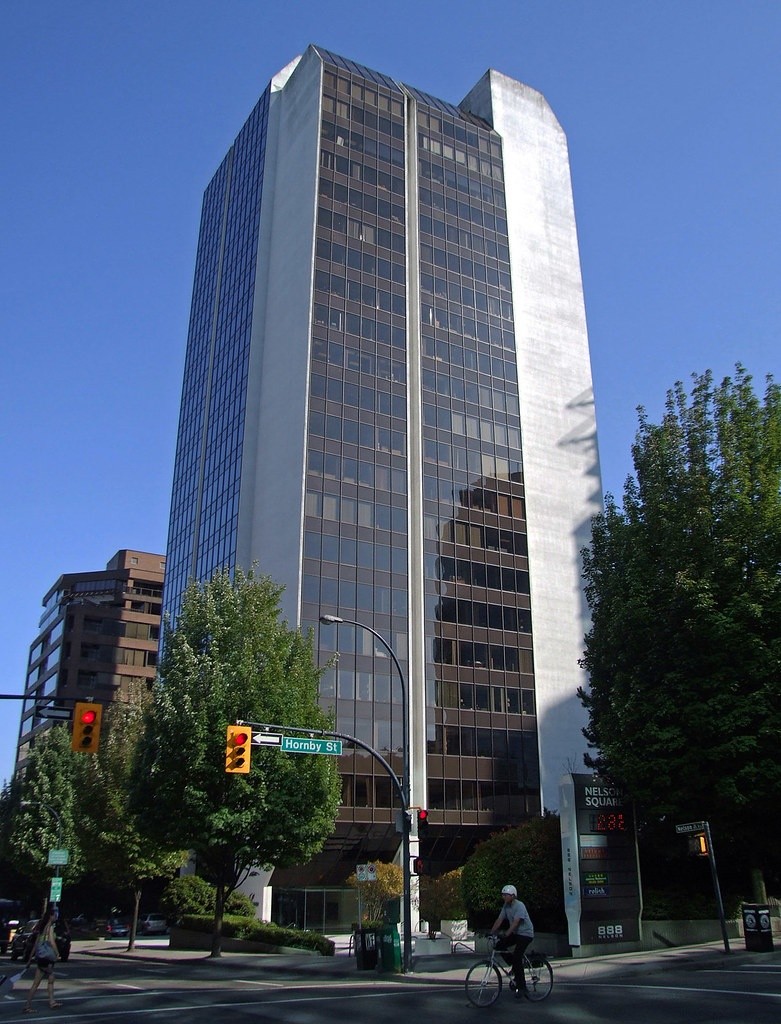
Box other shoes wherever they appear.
[48,1001,64,1010]
[22,1008,40,1018]
[509,970,515,976]
[515,989,529,998]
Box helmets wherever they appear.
[501,885,517,898]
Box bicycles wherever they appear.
[466,931,553,1009]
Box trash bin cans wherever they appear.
[352,928,376,971]
[741,903,776,953]
[380,925,402,972]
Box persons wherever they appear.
[484,885,535,998]
[23,909,64,1014]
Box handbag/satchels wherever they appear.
[35,940,58,962]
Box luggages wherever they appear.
[0,968,28,996]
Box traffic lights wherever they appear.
[413,857,431,875]
[72,702,102,752]
[225,726,250,773]
[417,810,428,839]
[695,834,708,856]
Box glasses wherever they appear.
[502,894,511,897]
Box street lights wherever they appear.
[19,801,62,910]
[319,614,412,973]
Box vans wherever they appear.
[140,912,170,935]
[0,899,23,953]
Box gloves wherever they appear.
[497,933,508,946]
[485,930,493,939]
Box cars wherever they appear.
[10,919,72,960]
[104,917,130,938]
[71,913,91,927]
